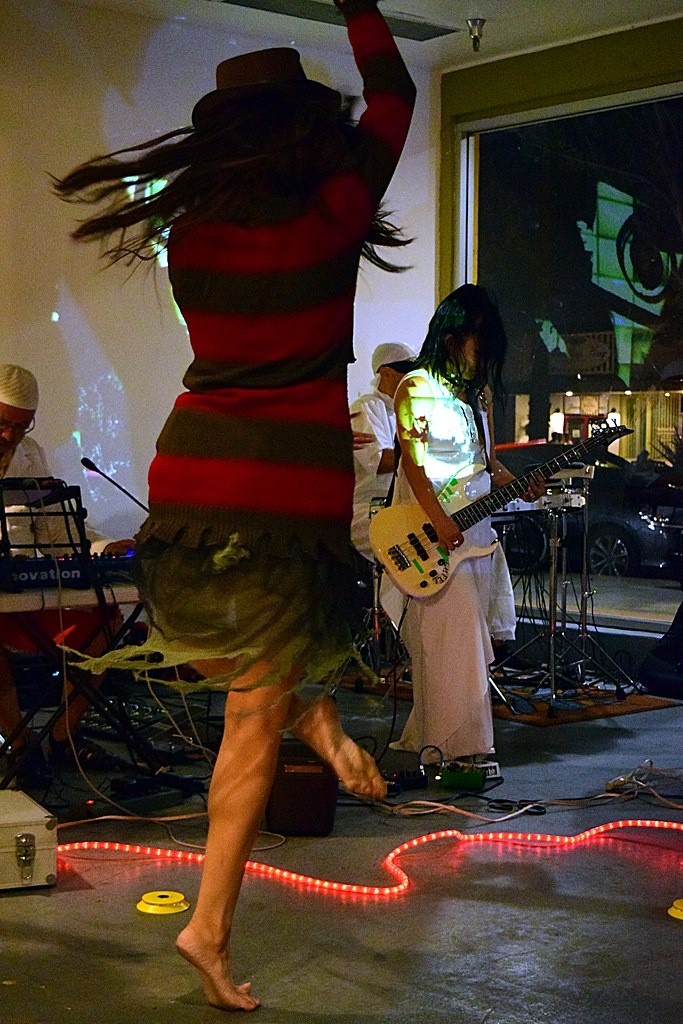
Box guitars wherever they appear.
[367,416,636,601]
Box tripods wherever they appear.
[327,566,414,698]
[492,480,649,719]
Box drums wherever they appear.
[368,496,387,524]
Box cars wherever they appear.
[495,440,683,577]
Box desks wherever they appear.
[0,587,210,799]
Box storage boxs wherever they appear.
[0,790,59,890]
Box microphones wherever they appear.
[80,456,149,512]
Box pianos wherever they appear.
[0,553,169,807]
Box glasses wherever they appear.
[0,418,36,434]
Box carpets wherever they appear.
[315,663,683,728]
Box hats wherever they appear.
[373,343,418,375]
[192,47,342,127]
[0,364,39,410]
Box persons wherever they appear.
[350,284,545,767]
[45,0,419,1011]
[0,364,135,784]
